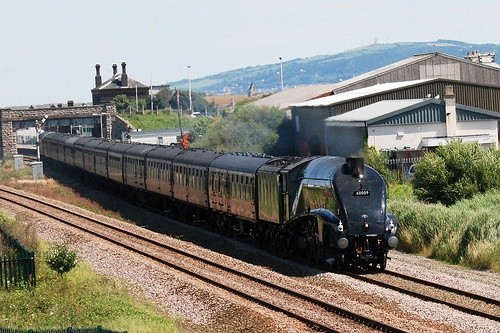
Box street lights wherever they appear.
[186,66,192,109]
[279,57,284,90]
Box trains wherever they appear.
[38,131,400,275]
